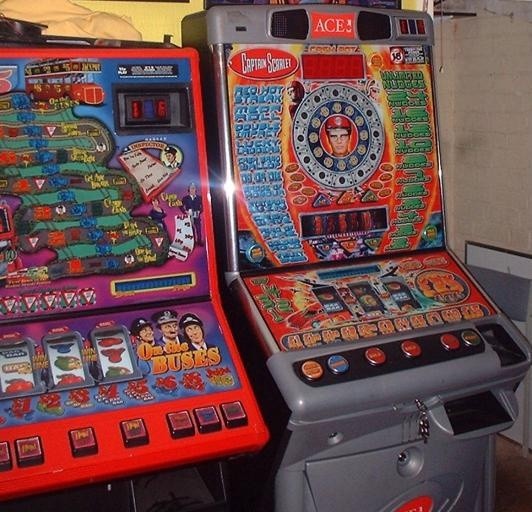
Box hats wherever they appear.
[133,309,206,330]
[164,144,179,153]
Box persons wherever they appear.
[131,317,162,346]
[150,196,172,253]
[165,147,181,168]
[152,309,188,353]
[179,314,215,356]
[180,182,205,246]
[324,114,354,157]
